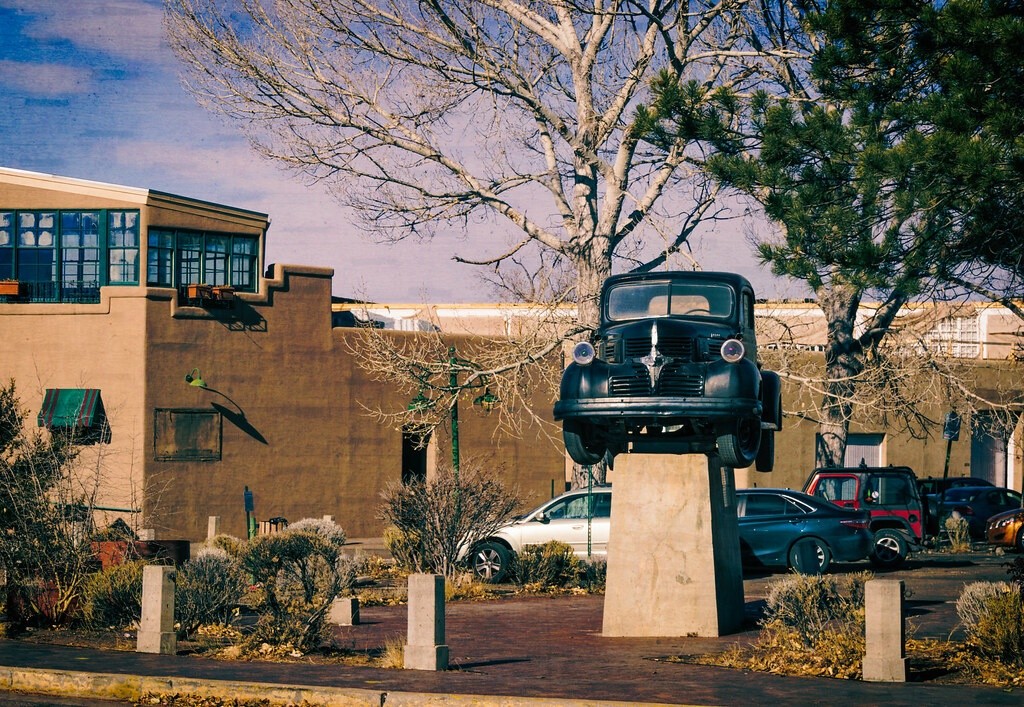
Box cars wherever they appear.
[916,476,1024,550]
[736,489,872,575]
[552,269,785,473]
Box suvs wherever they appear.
[471,482,613,583]
[800,465,939,571]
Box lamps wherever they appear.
[185,367,208,388]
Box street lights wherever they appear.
[407,345,502,525]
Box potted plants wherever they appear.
[211,283,235,301]
[0,278,28,296]
[187,282,211,299]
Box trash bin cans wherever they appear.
[258,517,288,534]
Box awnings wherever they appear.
[37,389,101,427]
